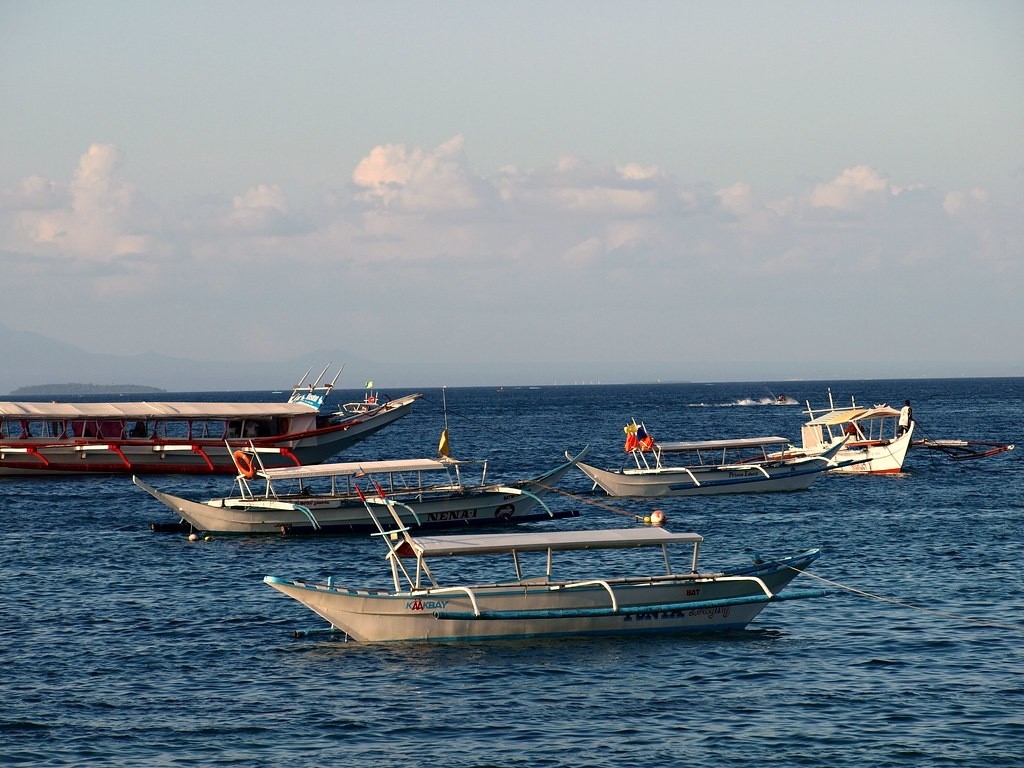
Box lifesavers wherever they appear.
[233,451,255,480]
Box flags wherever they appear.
[366,382,373,389]
[438,428,450,456]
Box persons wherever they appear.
[777,392,784,401]
[897,399,913,439]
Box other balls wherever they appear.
[651,511,667,523]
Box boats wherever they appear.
[132,387,592,540]
[264,477,823,651]
[564,386,916,503]
[1,359,425,477]
